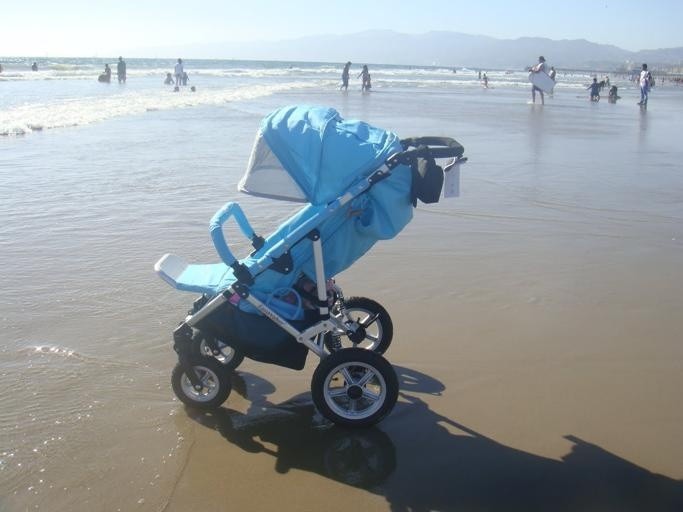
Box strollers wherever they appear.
[153,103,463,427]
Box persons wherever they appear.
[162,72,174,85]
[181,71,189,85]
[356,63,370,91]
[482,73,488,88]
[604,74,609,86]
[608,84,618,97]
[338,60,351,90]
[29,62,38,70]
[527,54,545,105]
[585,77,600,102]
[599,80,606,86]
[635,62,652,105]
[116,55,127,84]
[546,65,555,96]
[103,62,111,81]
[174,57,183,86]
